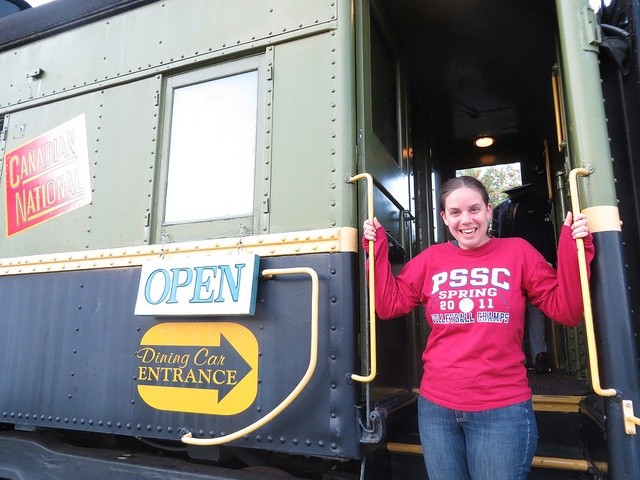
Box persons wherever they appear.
[362,176,595,480]
[491,182,558,375]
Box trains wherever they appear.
[0,0,640,480]
